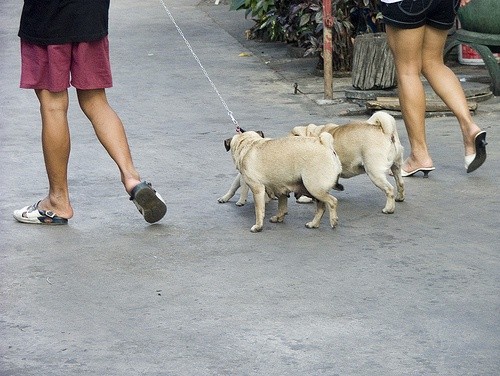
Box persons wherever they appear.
[14,0,167,224]
[365,0,488,178]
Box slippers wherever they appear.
[128,180,167,224]
[13,200,68,225]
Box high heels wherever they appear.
[464,130,488,173]
[389,163,436,177]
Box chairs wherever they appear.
[443,0,500,96]
